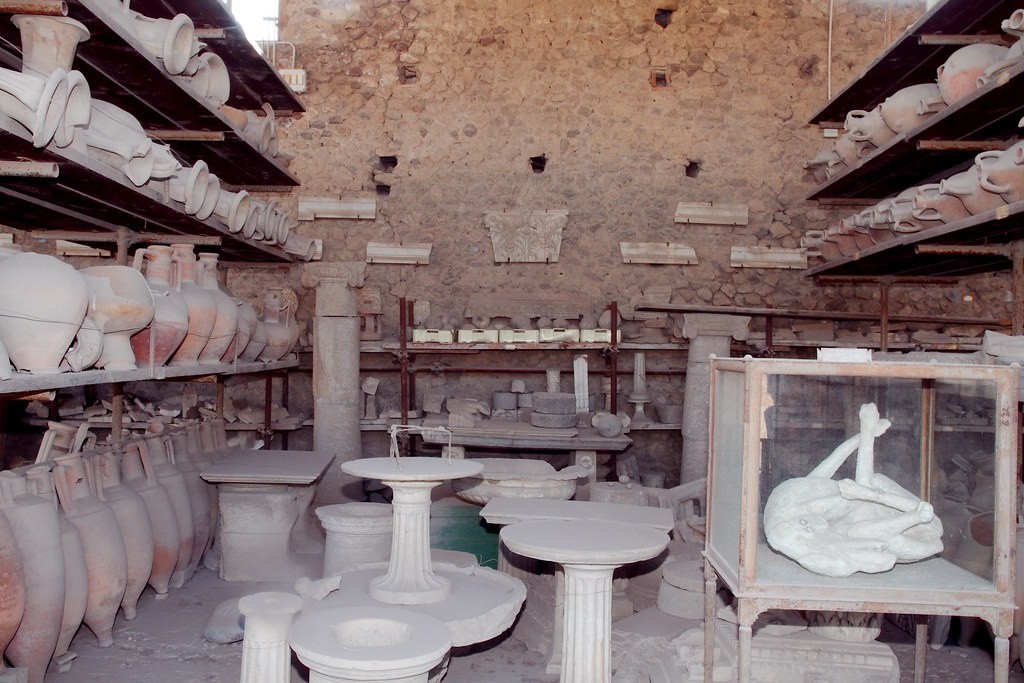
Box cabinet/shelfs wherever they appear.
[382,296,625,483]
[1,1,308,449]
[805,0,1023,286]
[699,352,1021,683]
